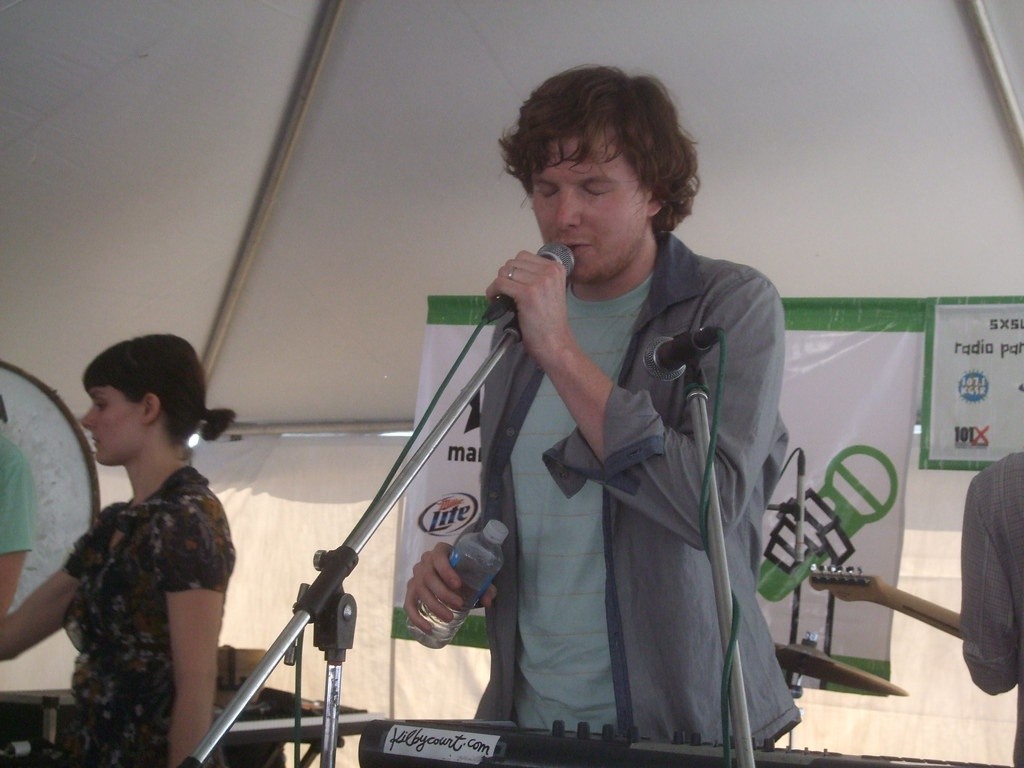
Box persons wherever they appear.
[960,450,1023,768]
[403,65,804,751]
[0,333,237,768]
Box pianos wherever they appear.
[356,716,1012,768]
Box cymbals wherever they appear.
[750,641,910,699]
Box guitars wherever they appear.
[806,560,964,642]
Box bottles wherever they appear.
[406,519,509,649]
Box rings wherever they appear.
[506,266,517,279]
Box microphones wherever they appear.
[482,243,574,324]
[642,326,725,382]
